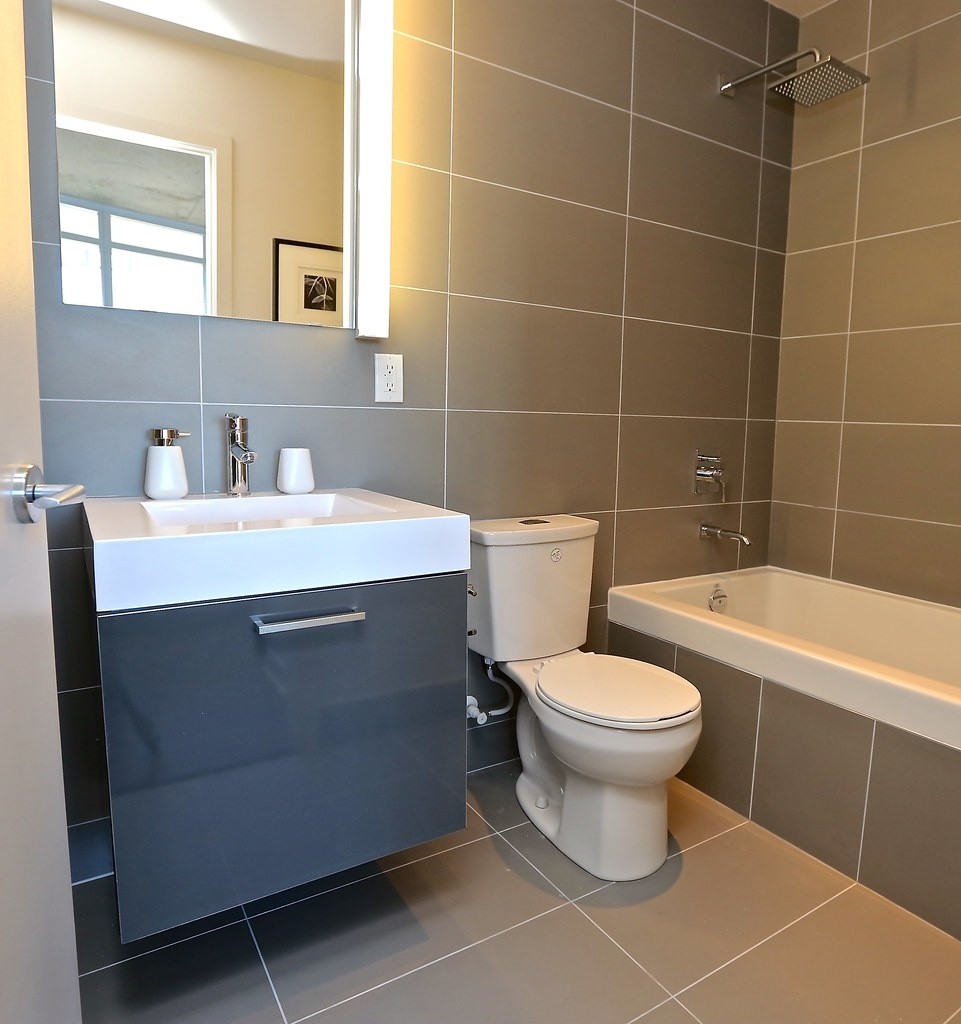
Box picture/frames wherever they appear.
[273,237,341,327]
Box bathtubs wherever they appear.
[605,565,961,945]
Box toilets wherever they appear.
[459,512,703,882]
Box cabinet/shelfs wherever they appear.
[97,575,468,945]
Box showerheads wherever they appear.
[767,55,872,108]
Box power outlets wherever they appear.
[375,352,404,404]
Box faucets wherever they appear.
[223,412,258,497]
[698,521,752,547]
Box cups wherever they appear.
[277,448,315,494]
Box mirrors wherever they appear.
[52,1,354,329]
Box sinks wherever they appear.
[143,427,192,500]
[139,494,398,556]
